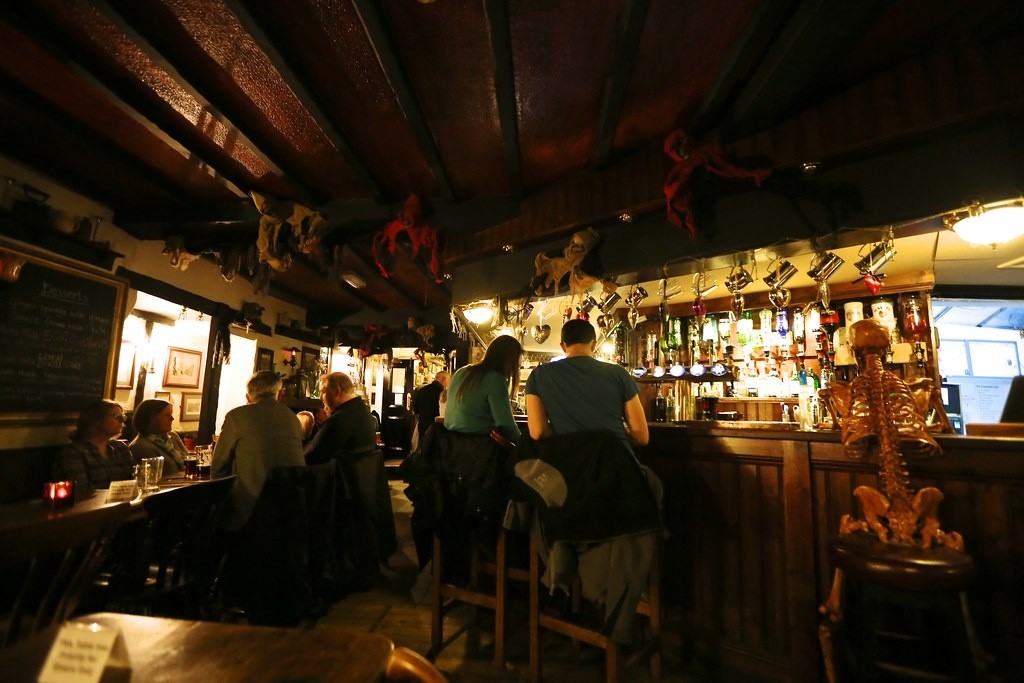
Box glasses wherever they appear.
[107,413,123,423]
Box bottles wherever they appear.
[645,295,927,371]
[655,385,676,422]
[699,363,829,398]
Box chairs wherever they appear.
[0,476,236,646]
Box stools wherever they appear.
[429,422,662,683]
[827,533,977,683]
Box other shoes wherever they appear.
[440,583,462,606]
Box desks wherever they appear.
[0,473,236,528]
[0,611,395,683]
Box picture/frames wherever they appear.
[180,392,202,422]
[154,392,170,402]
[302,346,320,364]
[162,346,202,388]
[256,347,274,371]
[116,340,136,390]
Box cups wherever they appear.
[42,480,76,507]
[133,456,164,493]
[184,445,213,479]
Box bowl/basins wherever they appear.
[718,412,738,420]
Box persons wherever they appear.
[128,400,192,510]
[57,398,149,557]
[411,370,450,448]
[526,319,664,619]
[442,334,524,571]
[303,372,378,466]
[209,370,307,530]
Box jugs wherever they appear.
[656,278,686,303]
[575,297,597,314]
[625,281,648,310]
[807,251,844,286]
[724,266,755,294]
[853,239,897,274]
[597,290,621,314]
[762,259,798,291]
[690,271,719,297]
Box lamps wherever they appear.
[283,347,301,370]
